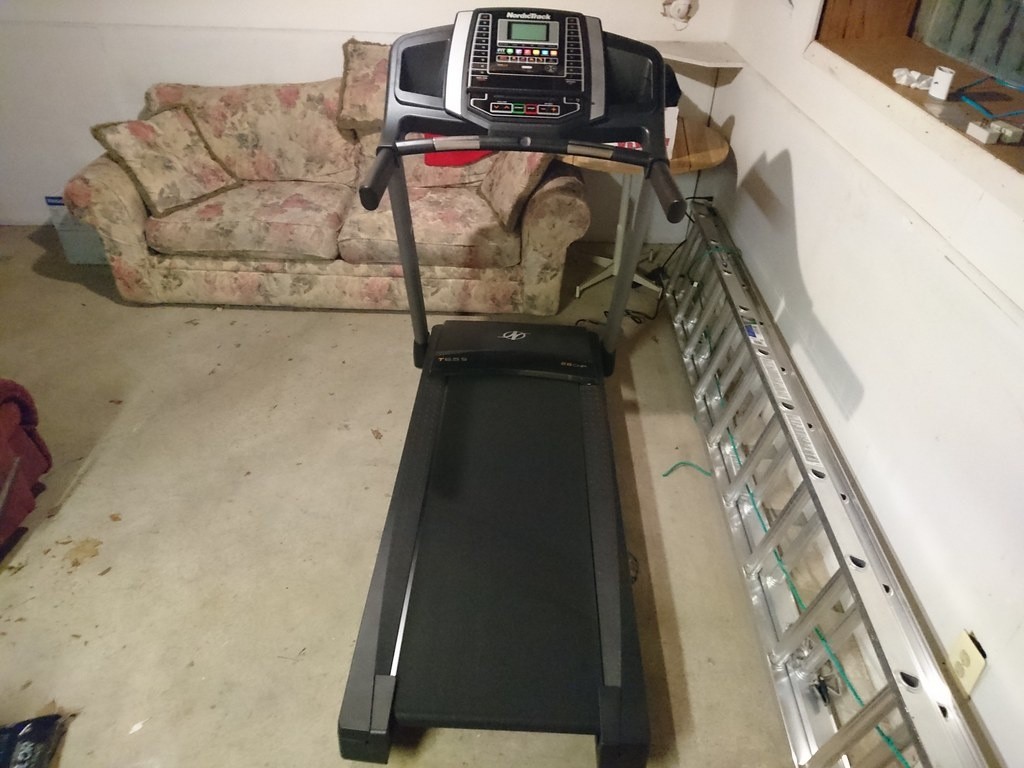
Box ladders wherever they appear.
[656,195,998,768]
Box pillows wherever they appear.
[88,102,243,217]
[339,37,390,129]
[480,150,556,234]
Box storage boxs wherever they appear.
[44,190,107,265]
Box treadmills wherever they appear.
[336,4,690,768]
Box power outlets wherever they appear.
[950,629,984,701]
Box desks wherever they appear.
[555,114,729,300]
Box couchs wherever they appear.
[65,79,590,316]
